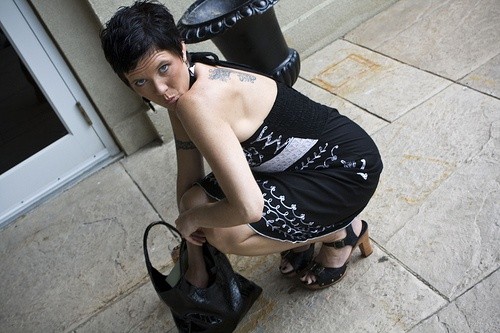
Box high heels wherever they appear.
[278,241,315,279]
[299,217,373,290]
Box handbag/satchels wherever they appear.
[143,219,263,333]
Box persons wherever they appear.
[101,1,383,289]
